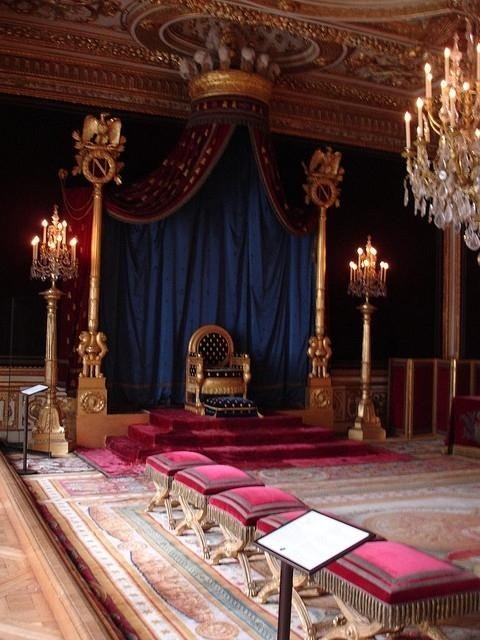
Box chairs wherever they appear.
[184,323,252,415]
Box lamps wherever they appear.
[347,234,391,443]
[393,0,480,265]
[30,203,81,459]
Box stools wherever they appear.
[145,448,217,530]
[255,510,387,639]
[309,539,478,638]
[203,396,258,418]
[168,463,266,560]
[206,485,309,599]
